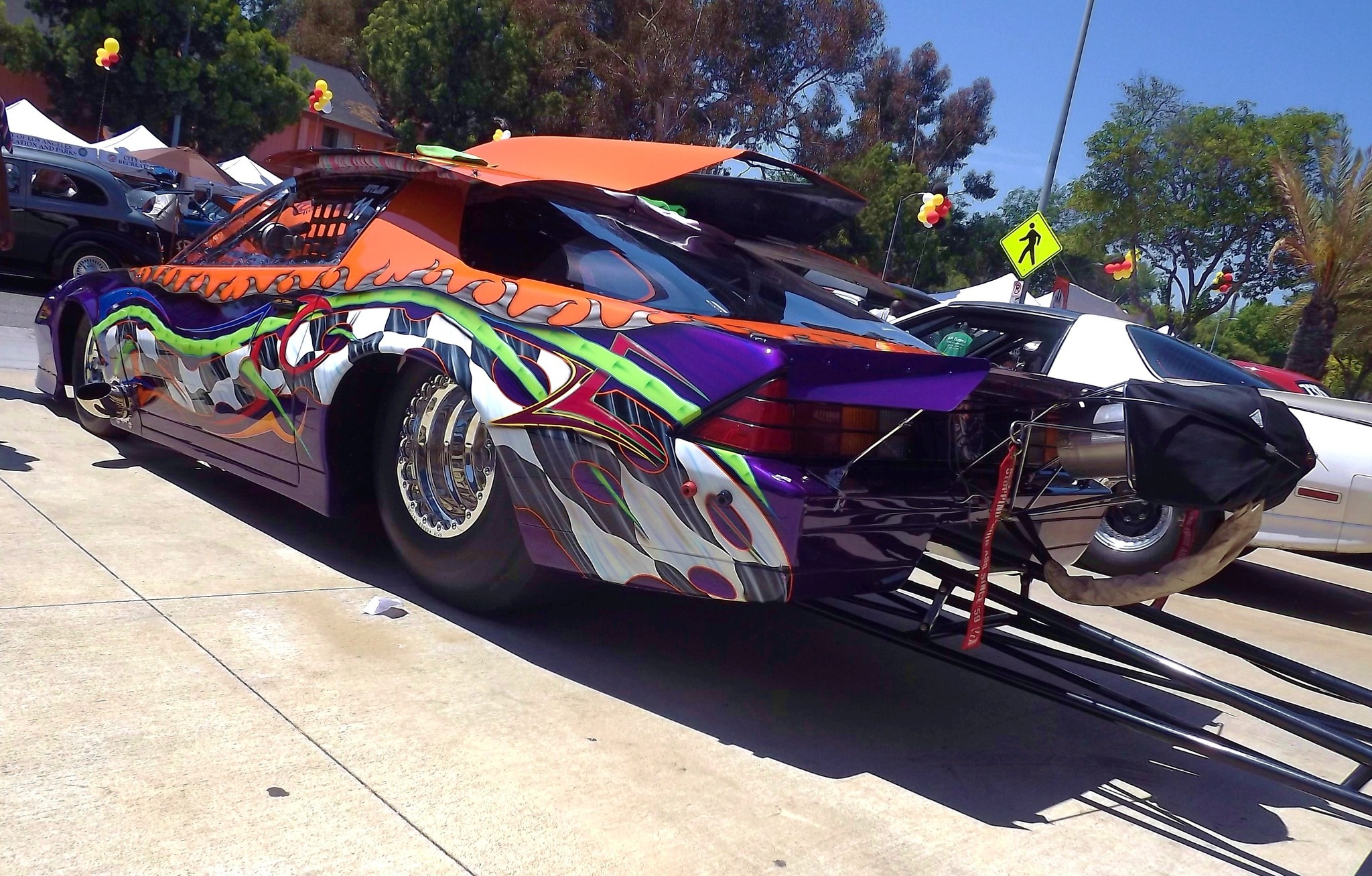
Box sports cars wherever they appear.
[800,270,1371,578]
[35,134,1316,619]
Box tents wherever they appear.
[5,99,290,197]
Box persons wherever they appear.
[142,194,182,235]
[937,325,973,356]
[0,98,13,251]
[869,300,904,322]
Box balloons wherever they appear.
[1212,265,1233,292]
[308,79,333,114]
[1104,252,1133,281]
[493,129,511,141]
[917,182,952,228]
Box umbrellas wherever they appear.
[121,147,244,260]
[95,37,120,70]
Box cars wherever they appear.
[0,144,244,287]
